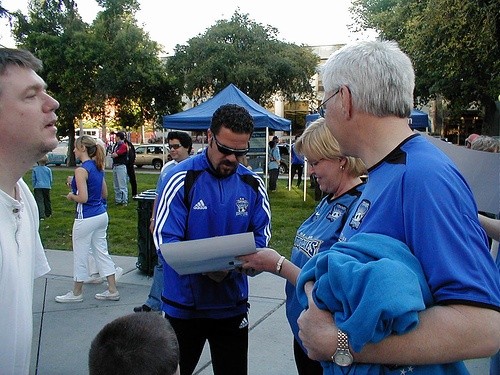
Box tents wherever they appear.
[162,83,292,192]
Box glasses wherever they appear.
[212,133,250,156]
[169,144,182,149]
[317,90,352,118]
[307,157,324,168]
[465,139,471,147]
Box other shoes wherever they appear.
[95,289,120,300]
[114,267,123,280]
[134,304,163,315]
[83,276,104,283]
[55,288,84,302]
[114,201,127,207]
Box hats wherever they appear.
[269,141,275,148]
[273,136,279,139]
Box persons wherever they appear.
[110,132,129,205]
[268,141,280,190]
[66,137,105,284]
[118,131,137,197]
[296,40,500,375]
[32,154,52,220]
[133,130,194,314]
[154,103,272,375]
[89,313,181,375]
[55,136,120,303]
[133,131,192,312]
[0,48,59,375]
[287,134,305,188]
[235,119,370,375]
[272,136,279,152]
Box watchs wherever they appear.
[330,330,353,367]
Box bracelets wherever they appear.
[149,218,155,221]
[275,256,285,275]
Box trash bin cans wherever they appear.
[130,186,159,278]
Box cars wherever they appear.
[36,144,82,166]
[134,145,174,170]
[277,144,296,175]
